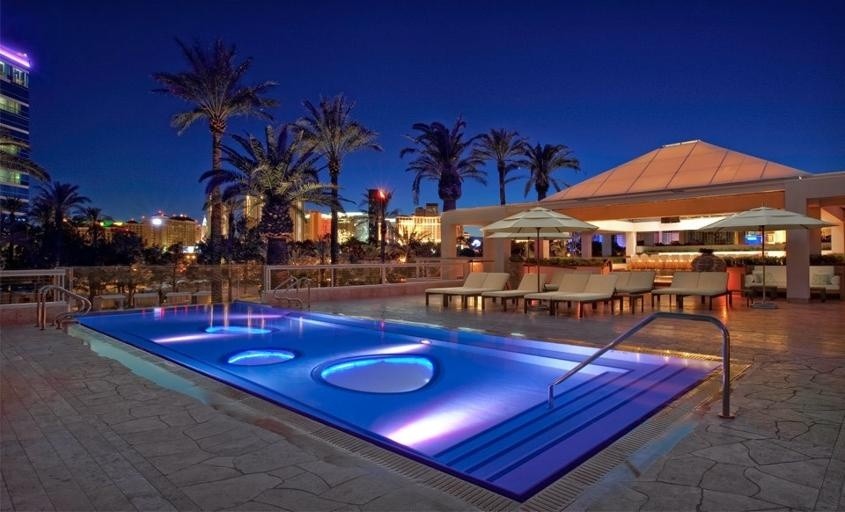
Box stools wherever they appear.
[728,289,755,308]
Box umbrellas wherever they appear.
[479,205,599,306]
[486,232,571,273]
[700,202,841,302]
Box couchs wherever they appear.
[744,263,841,303]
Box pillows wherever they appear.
[752,269,769,282]
[811,273,830,285]
